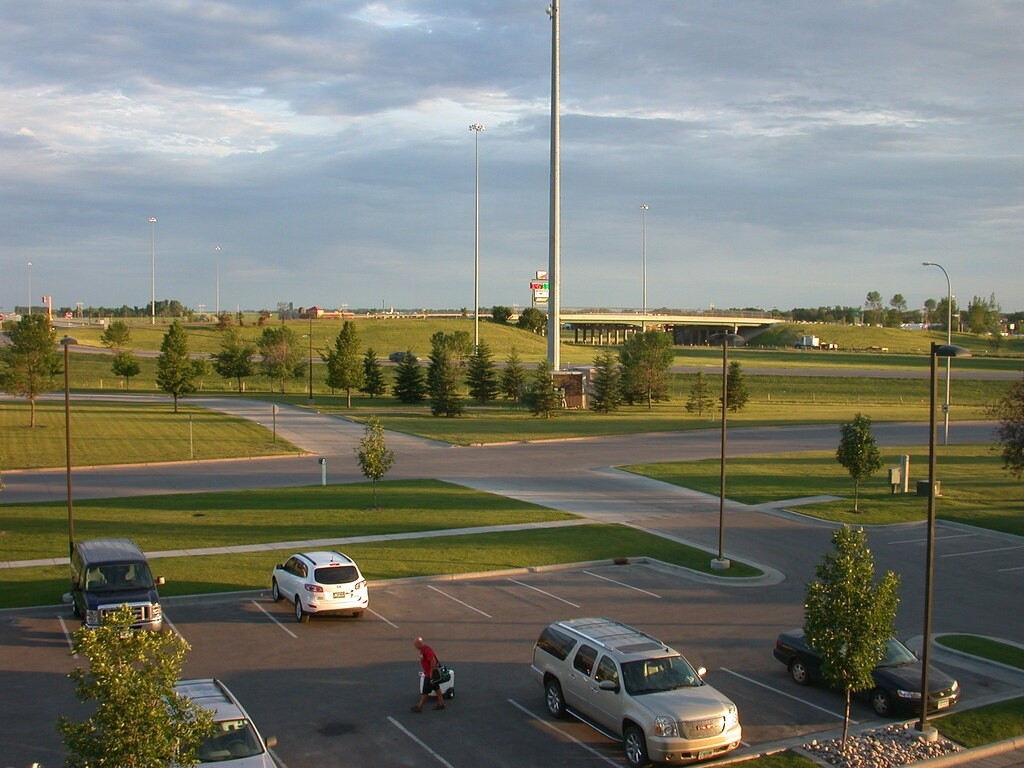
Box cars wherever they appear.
[775,622,962,715]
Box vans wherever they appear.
[70,537,165,643]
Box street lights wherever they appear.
[641,203,660,325]
[215,246,224,320]
[708,328,745,572]
[59,334,80,560]
[469,121,485,359]
[147,216,162,327]
[906,342,972,743]
[27,261,33,319]
[921,261,954,443]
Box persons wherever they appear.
[411,637,447,712]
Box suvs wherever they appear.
[532,618,743,766]
[159,678,279,768]
[271,549,369,624]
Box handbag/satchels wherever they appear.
[431,665,451,685]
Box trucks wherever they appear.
[793,334,839,352]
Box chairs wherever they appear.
[644,657,677,693]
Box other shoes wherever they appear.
[432,703,446,709]
[410,706,423,712]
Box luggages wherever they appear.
[417,669,455,698]
